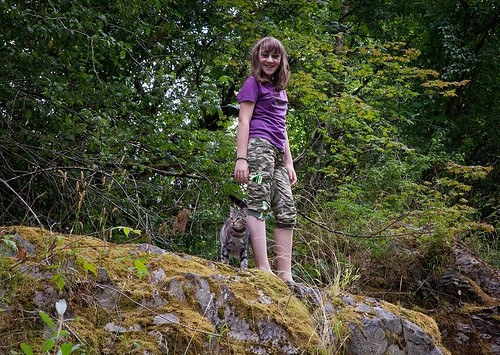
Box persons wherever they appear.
[234,36,297,282]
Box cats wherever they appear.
[219,207,250,270]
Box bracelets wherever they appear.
[235,158,248,161]
